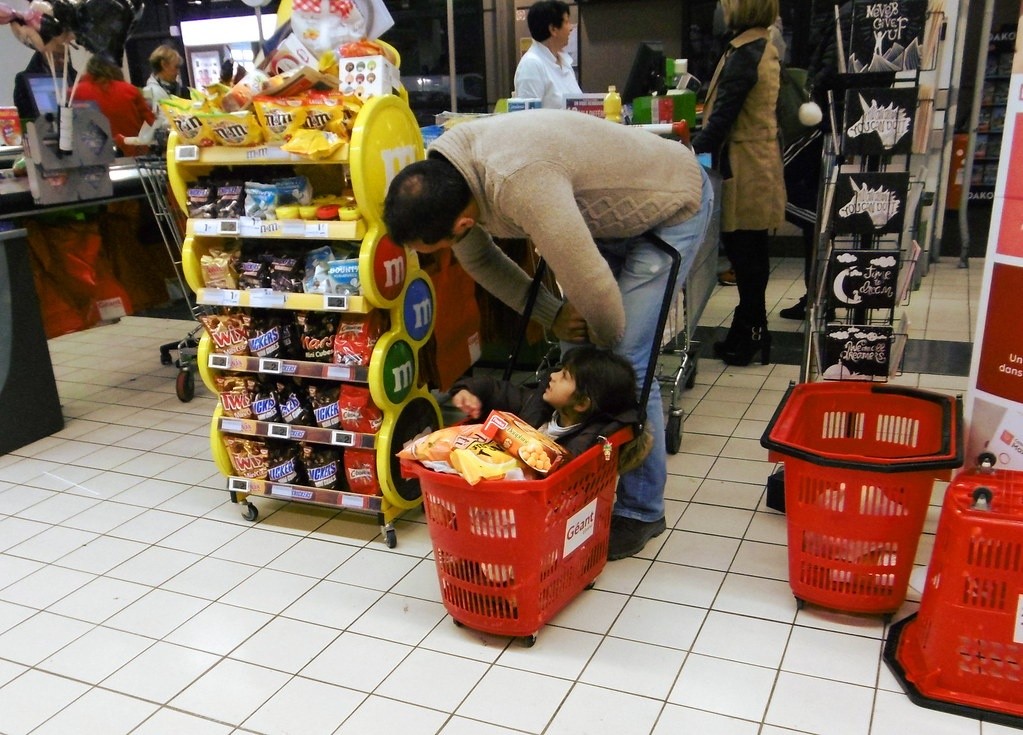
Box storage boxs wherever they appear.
[481,410,568,477]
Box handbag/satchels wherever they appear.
[776,60,822,145]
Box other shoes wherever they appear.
[718,268,737,285]
[779,294,835,322]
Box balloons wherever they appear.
[0,0,145,54]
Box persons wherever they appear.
[382,108,715,561]
[514,0,584,94]
[143,44,184,99]
[13,44,77,118]
[221,60,248,85]
[447,348,655,475]
[67,54,156,137]
[690,0,786,366]
[780,0,876,320]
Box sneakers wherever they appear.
[606,516,666,560]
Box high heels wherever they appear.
[722,307,772,366]
[713,306,741,353]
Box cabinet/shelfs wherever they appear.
[167,91,445,546]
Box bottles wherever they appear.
[603,86,621,124]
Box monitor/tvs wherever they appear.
[621,41,668,104]
[12,70,81,131]
